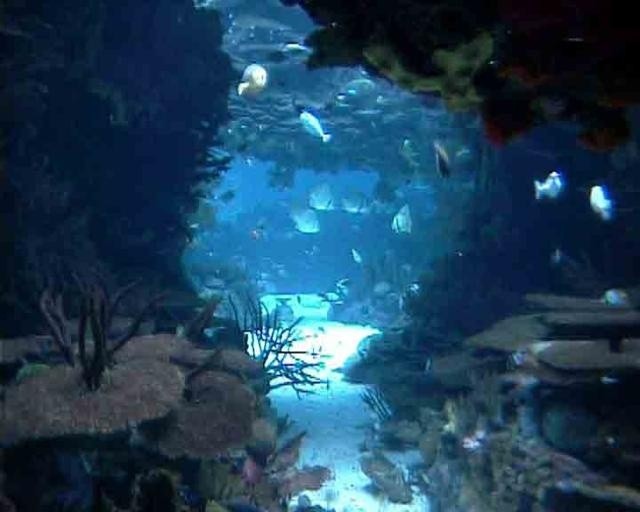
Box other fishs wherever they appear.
[175,5,628,505]
